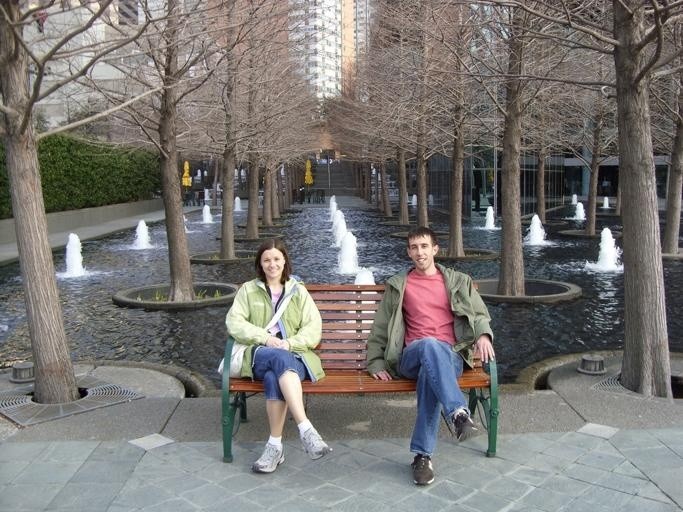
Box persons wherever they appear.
[215,238,332,474]
[364,226,495,487]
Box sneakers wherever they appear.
[412,454,435,486]
[452,411,478,442]
[252,444,285,473]
[301,427,333,461]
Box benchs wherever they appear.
[222,284,499,462]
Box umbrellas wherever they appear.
[302,159,313,183]
[181,159,191,186]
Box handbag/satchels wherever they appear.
[219,341,247,379]
